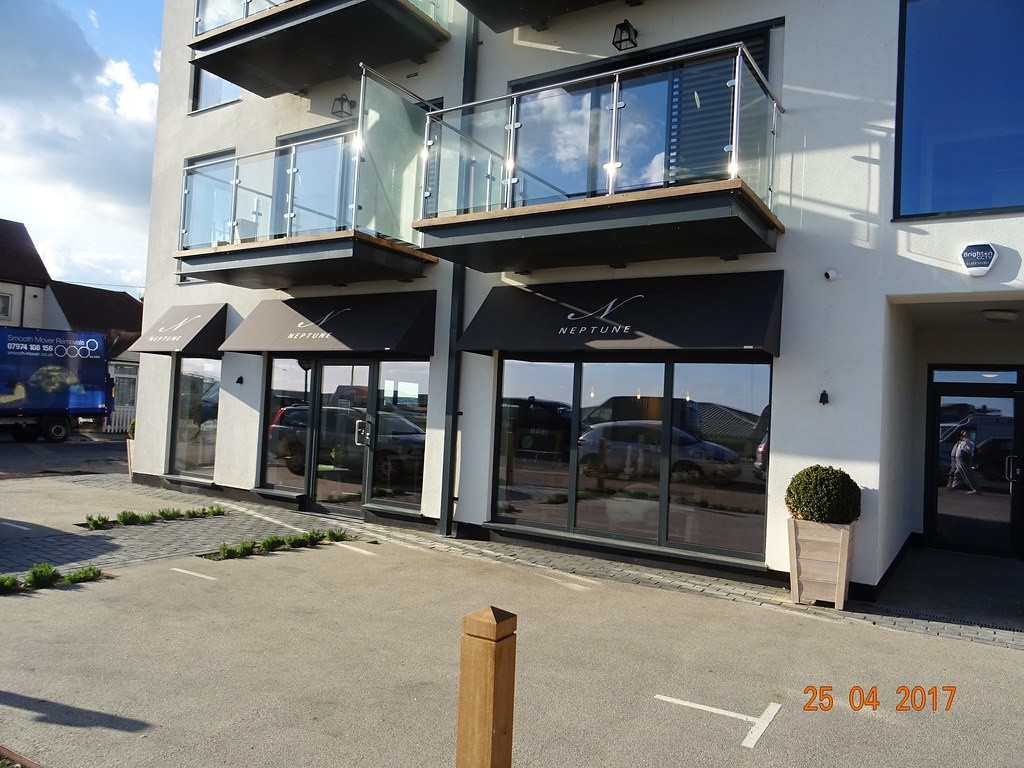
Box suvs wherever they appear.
[578,421,742,483]
[268,401,426,485]
[199,380,303,421]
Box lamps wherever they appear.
[321,90,358,122]
[609,16,640,56]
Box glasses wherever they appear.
[963,434,968,437]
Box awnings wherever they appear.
[126,301,228,353]
[216,289,438,356]
[453,268,787,358]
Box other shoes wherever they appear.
[968,489,979,494]
[944,485,952,492]
[951,487,961,492]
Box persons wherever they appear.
[944,430,980,495]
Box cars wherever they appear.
[753,432,768,480]
[975,437,1013,469]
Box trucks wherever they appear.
[582,397,702,447]
[0,325,115,443]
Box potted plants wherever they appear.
[778,461,866,621]
